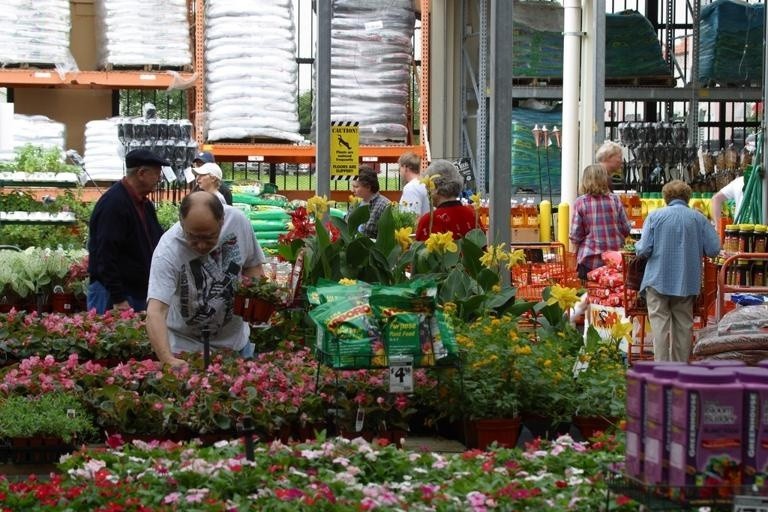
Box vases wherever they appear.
[51,291,72,314]
[463,414,520,448]
[240,293,254,321]
[523,414,570,439]
[0,287,21,313]
[438,412,461,439]
[232,292,244,315]
[24,292,49,315]
[76,294,88,312]
[251,296,272,323]
[576,414,619,440]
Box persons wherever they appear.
[144,191,267,369]
[397,152,435,217]
[344,164,392,242]
[417,159,487,251]
[569,164,630,285]
[86,150,172,314]
[597,139,624,193]
[192,150,233,206]
[710,175,747,236]
[634,180,720,363]
[191,162,226,206]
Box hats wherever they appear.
[193,152,215,164]
[191,163,222,181]
[126,150,171,168]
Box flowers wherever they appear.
[233,275,256,295]
[435,314,628,419]
[0,350,434,464]
[254,281,289,302]
[0,246,89,297]
[243,273,269,294]
[0,303,158,362]
[280,174,577,318]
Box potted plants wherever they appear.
[0,189,82,220]
[0,214,89,250]
[2,144,81,186]
[0,395,94,459]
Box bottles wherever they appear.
[723,225,732,252]
[736,260,749,287]
[727,260,738,285]
[729,225,740,252]
[738,224,755,252]
[753,224,766,253]
[752,261,763,286]
[716,259,729,284]
